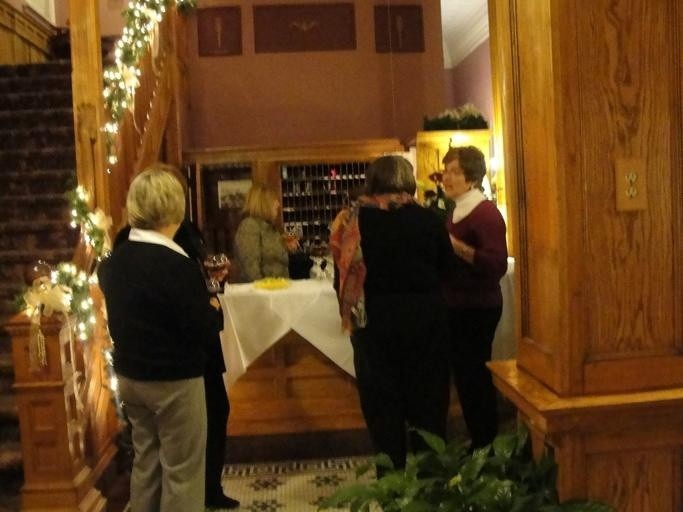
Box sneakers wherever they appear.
[204,485,240,511]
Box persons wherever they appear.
[440,146,508,461]
[327,155,454,479]
[94,167,221,512]
[111,163,240,510]
[230,182,300,282]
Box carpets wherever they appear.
[124,453,383,511]
